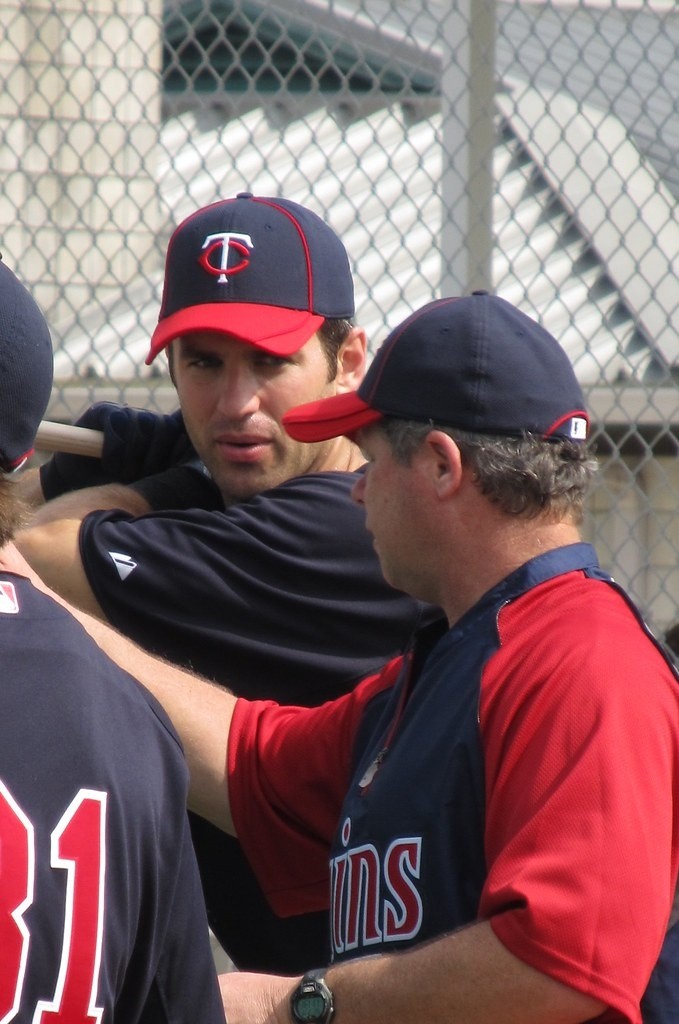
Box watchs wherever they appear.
[289,966,335,1024]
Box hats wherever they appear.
[144,192,353,365]
[282,288,591,444]
[0,252,54,473]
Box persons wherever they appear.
[14,190,436,983]
[0,294,679,1024]
[0,263,228,1024]
